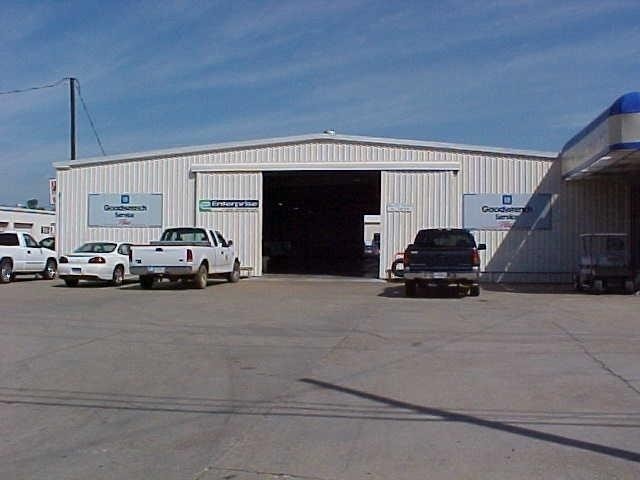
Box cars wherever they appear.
[57,240,144,288]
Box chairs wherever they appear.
[424,239,436,245]
[456,239,468,246]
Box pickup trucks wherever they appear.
[0,232,58,284]
[129,226,243,292]
[402,226,487,298]
